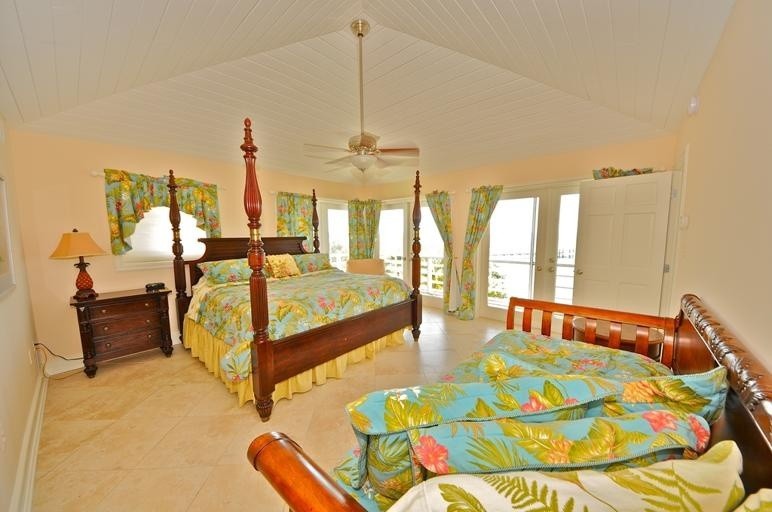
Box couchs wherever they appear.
[243,292,772,511]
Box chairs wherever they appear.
[346,259,385,276]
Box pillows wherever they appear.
[197,252,331,288]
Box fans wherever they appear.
[300,19,420,177]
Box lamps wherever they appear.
[348,154,374,175]
[48,228,107,300]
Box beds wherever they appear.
[166,116,422,423]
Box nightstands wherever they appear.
[68,286,172,379]
